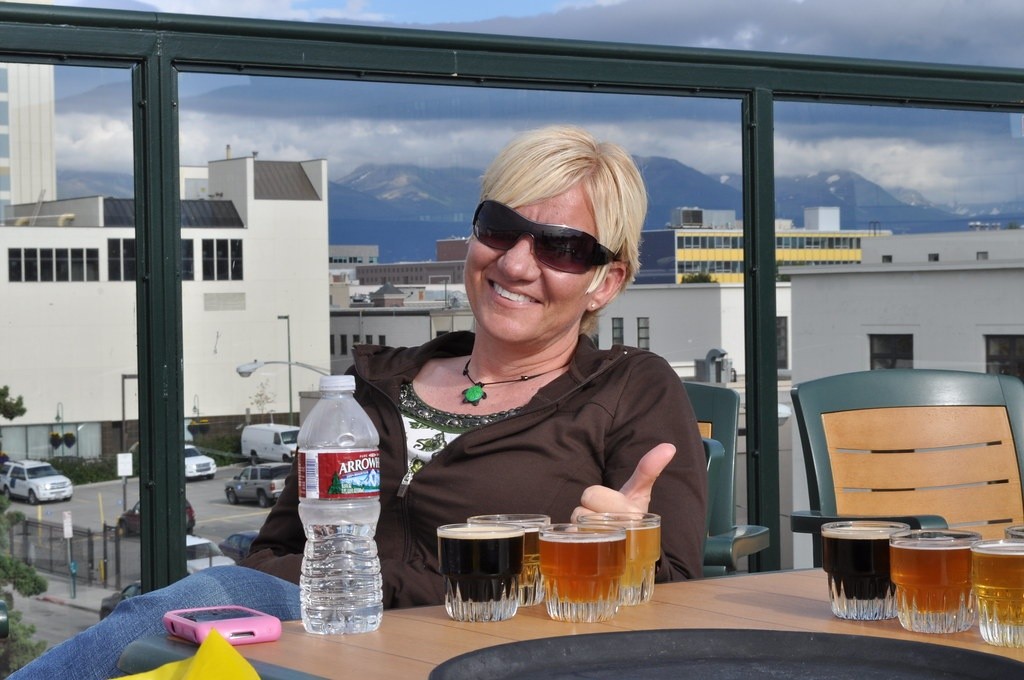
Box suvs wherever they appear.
[0,460,73,503]
[223,463,295,507]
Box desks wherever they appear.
[116,568,1024,680]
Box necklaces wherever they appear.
[462,358,572,405]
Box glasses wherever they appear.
[473,199,619,274]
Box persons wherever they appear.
[0,125,707,680]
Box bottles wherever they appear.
[297,374,385,635]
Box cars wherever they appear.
[100,580,141,625]
[220,528,263,558]
[118,494,197,535]
[186,535,236,575]
[129,440,217,481]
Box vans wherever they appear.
[241,425,302,466]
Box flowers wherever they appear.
[190,418,209,425]
[49,432,74,439]
[0,451,8,458]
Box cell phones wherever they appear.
[163,605,282,644]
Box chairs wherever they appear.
[681,368,1024,579]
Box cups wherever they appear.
[467,513,551,607]
[1004,525,1024,537]
[970,538,1024,648]
[890,528,982,633]
[436,523,525,623]
[576,511,662,605]
[821,520,910,620]
[538,523,627,622]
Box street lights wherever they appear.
[120,373,138,510]
[55,402,66,458]
[277,314,295,424]
